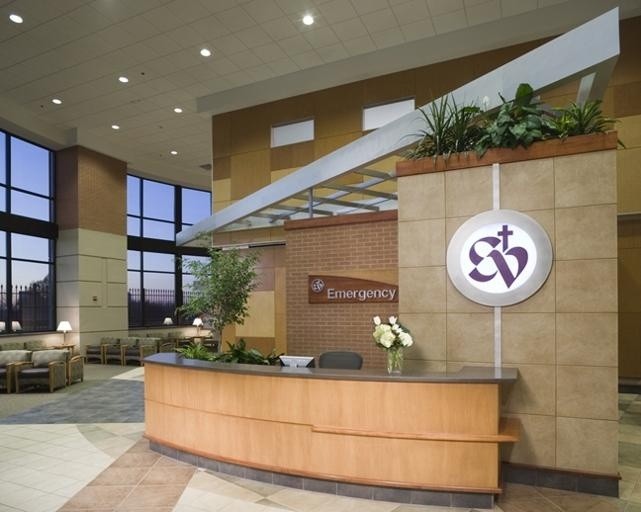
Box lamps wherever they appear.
[191,318,203,336]
[56,321,72,345]
[163,317,172,326]
[11,320,22,332]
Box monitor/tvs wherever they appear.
[279,355,316,368]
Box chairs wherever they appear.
[85,329,219,368]
[318,352,362,370]
[1,339,86,392]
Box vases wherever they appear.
[386,351,403,376]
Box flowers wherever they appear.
[371,315,416,369]
